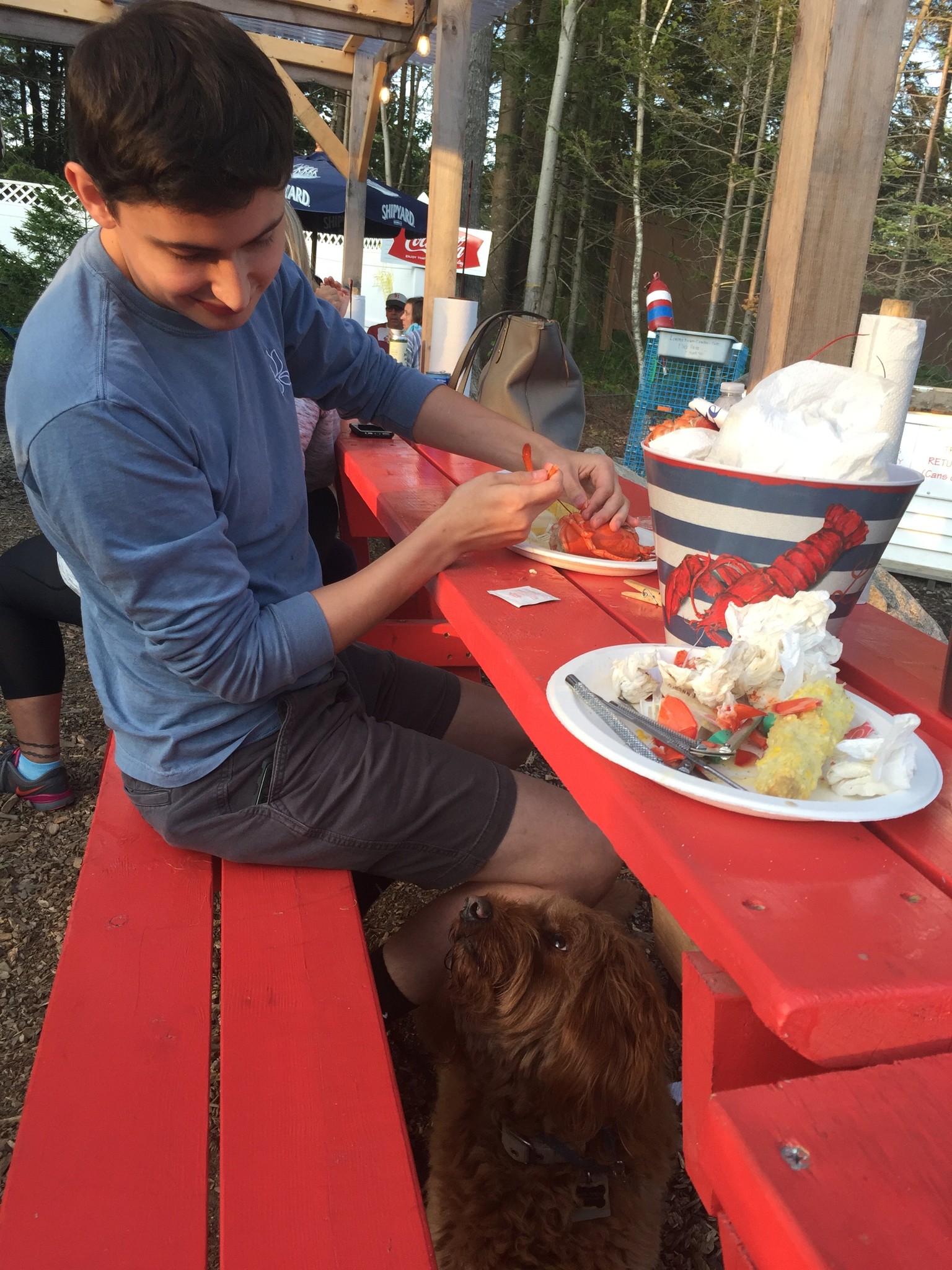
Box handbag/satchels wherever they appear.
[448,310,586,451]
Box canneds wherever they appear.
[389,335,408,367]
[426,371,452,386]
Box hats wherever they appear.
[386,294,408,309]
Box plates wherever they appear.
[495,469,657,577]
[545,643,944,823]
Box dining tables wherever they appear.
[332,418,952,1270]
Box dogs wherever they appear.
[427,892,679,1270]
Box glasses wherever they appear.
[385,307,404,313]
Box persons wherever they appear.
[5,1,637,1054]
[0,192,340,813]
[309,275,424,371]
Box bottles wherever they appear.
[712,381,746,411]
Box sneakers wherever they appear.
[0,747,74,812]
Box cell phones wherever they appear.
[349,423,395,439]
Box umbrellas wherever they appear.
[284,152,428,278]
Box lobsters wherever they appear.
[644,410,718,447]
[548,499,656,563]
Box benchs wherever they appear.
[1,733,436,1269]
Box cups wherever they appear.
[639,438,924,644]
